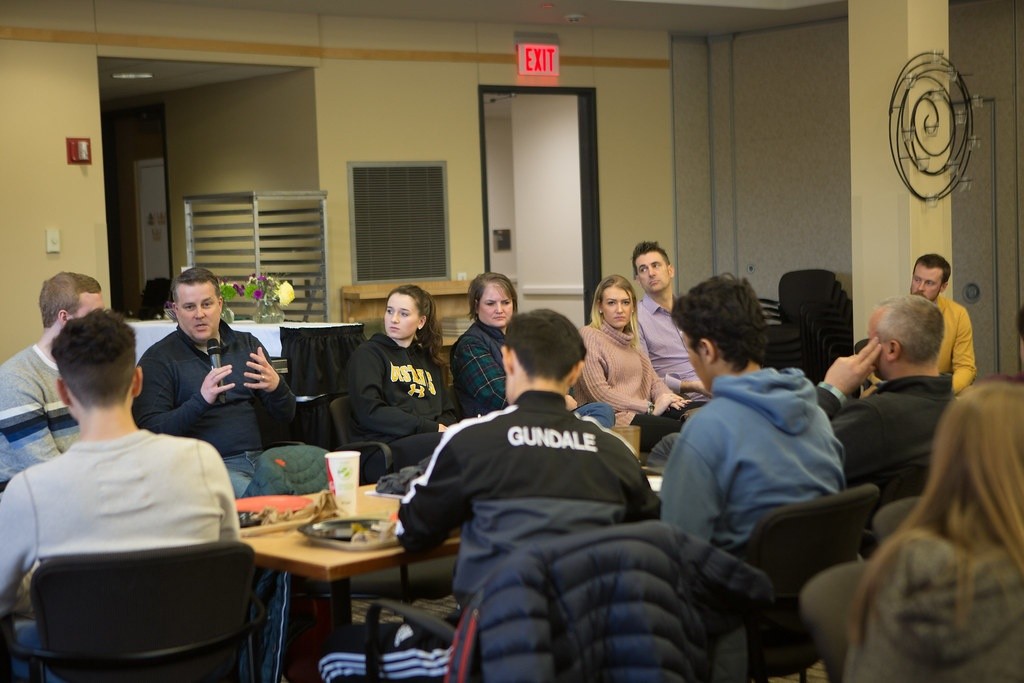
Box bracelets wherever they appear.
[648,402,654,415]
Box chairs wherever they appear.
[0,270,923,683]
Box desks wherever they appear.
[125,320,361,445]
[238,458,668,683]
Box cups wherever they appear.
[611,424,640,459]
[325,451,362,514]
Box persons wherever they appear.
[0,308,242,683]
[450,273,615,428]
[348,285,455,467]
[132,268,296,500]
[845,381,1024,683]
[990,308,1024,381]
[573,275,694,451]
[318,310,660,683]
[861,255,975,393]
[0,270,102,481]
[632,241,711,401]
[815,294,956,550]
[660,273,844,549]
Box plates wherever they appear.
[234,495,313,513]
[296,519,400,550]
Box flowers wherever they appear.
[217,278,244,302]
[232,273,295,306]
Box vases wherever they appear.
[252,300,286,325]
[221,298,235,324]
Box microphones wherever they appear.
[207,338,226,404]
